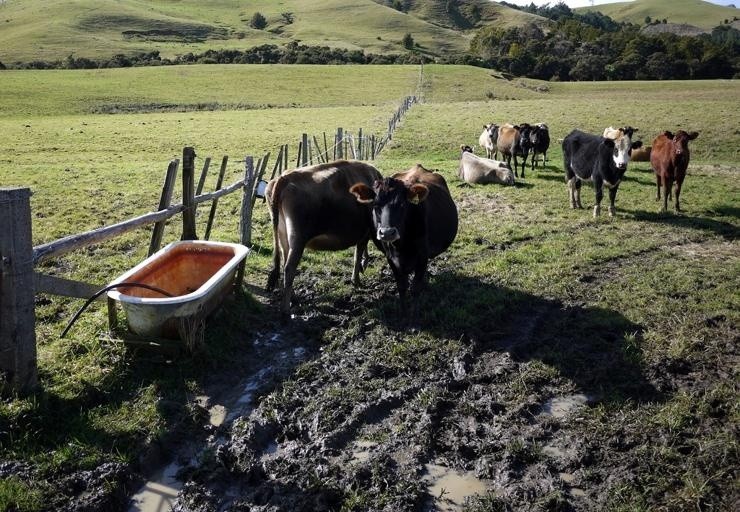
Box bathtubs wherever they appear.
[104,234,252,343]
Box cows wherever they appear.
[562,128,642,219]
[264,159,383,319]
[478,122,499,161]
[650,130,699,216]
[529,122,550,171]
[630,140,652,161]
[497,122,536,178]
[349,163,458,322]
[602,125,639,141]
[456,144,514,186]
